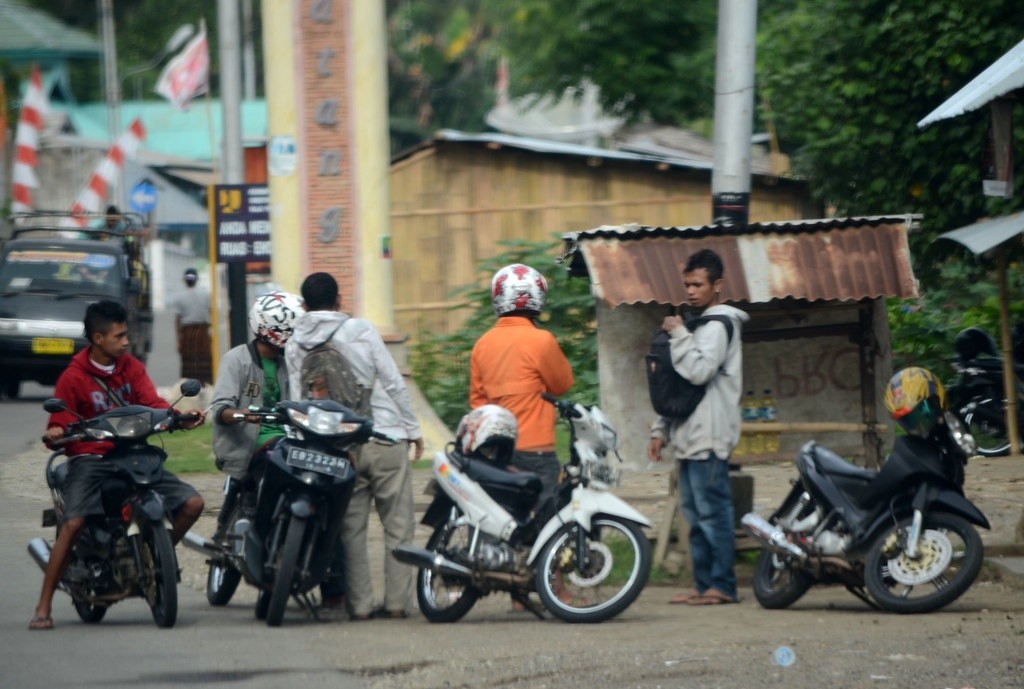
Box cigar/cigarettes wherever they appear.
[204,405,214,414]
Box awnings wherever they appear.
[930,211,1024,255]
[580,226,919,312]
[917,39,1024,131]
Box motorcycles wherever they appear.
[944,327,1024,457]
[28,378,202,630]
[739,392,991,616]
[391,391,654,624]
[180,398,401,626]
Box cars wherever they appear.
[1,211,154,403]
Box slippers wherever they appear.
[667,589,740,605]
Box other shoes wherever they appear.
[28,605,54,630]
[349,608,405,621]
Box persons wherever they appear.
[78,205,128,240]
[469,263,573,613]
[210,272,424,621]
[28,299,205,632]
[174,268,213,385]
[648,249,749,606]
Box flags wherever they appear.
[152,33,211,113]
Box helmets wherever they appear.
[491,262,549,316]
[455,403,518,467]
[954,327,999,361]
[248,291,306,355]
[884,367,945,432]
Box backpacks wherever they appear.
[295,319,373,442]
[644,314,733,420]
